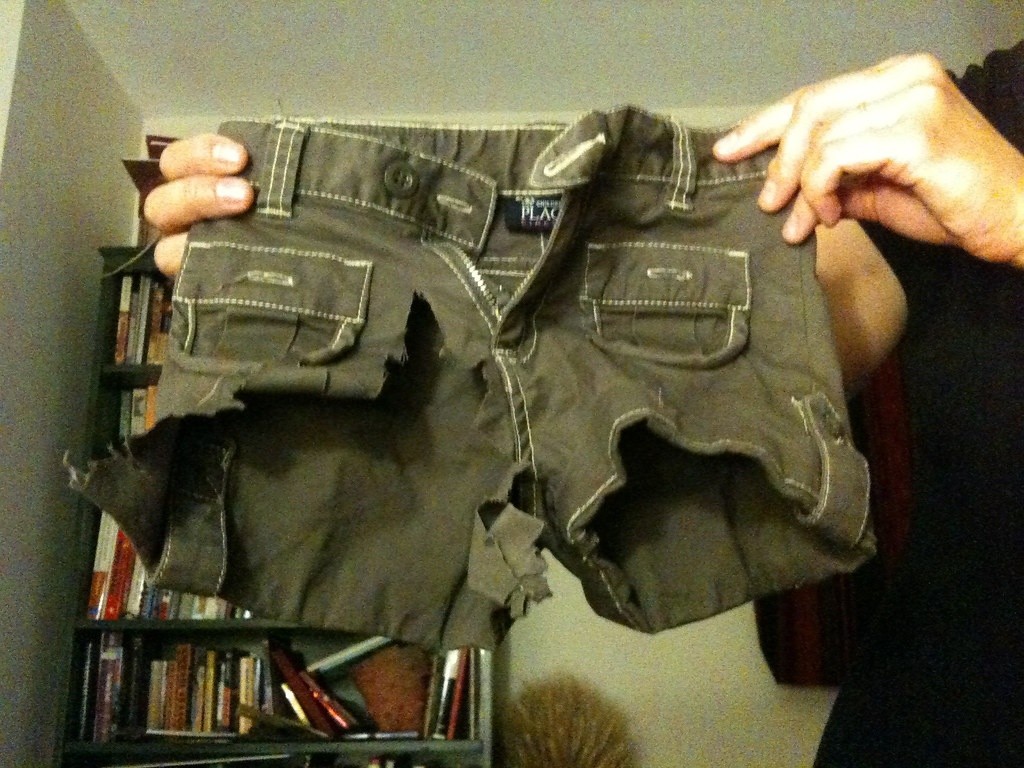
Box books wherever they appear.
[72,276,477,745]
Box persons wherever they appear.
[144,38,1023,766]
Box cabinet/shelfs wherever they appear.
[63,246,495,768]
